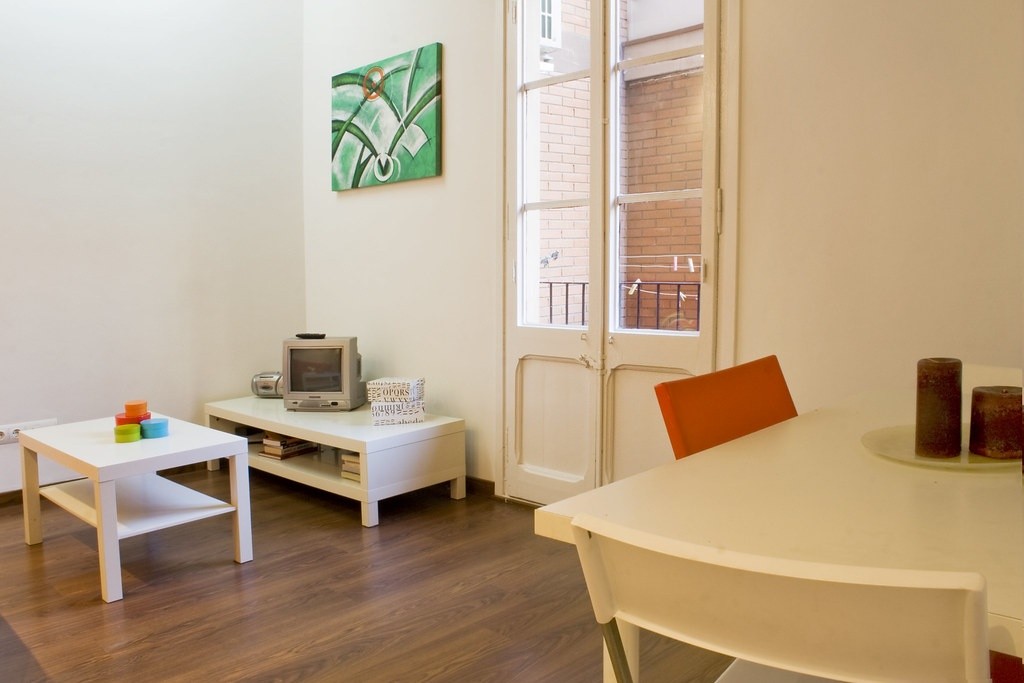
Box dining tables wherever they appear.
[532,376,1024,683]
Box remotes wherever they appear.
[296,333,326,339]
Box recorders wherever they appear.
[251,372,283,398]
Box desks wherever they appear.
[203,393,467,528]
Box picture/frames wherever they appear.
[329,41,443,192]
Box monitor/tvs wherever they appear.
[283,336,362,412]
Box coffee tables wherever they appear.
[18,411,255,604]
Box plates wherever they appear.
[860,421,1024,473]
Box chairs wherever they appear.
[570,511,996,683]
[653,354,800,463]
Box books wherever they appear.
[258,436,318,460]
[341,453,360,482]
